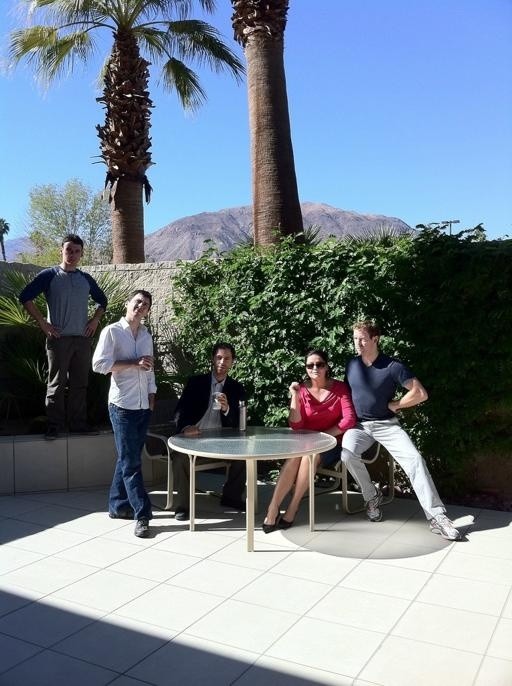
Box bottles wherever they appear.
[236,401,247,432]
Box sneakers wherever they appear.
[134,518,150,538]
[69,429,100,436]
[429,514,462,541]
[220,498,246,511]
[363,486,384,522]
[175,506,187,520]
[45,422,57,440]
[109,506,135,520]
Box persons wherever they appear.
[18,233,107,441]
[173,341,253,522]
[340,319,461,542]
[90,289,160,537]
[261,349,357,535]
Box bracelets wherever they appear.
[149,394,155,399]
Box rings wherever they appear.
[48,331,52,336]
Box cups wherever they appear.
[140,355,155,373]
[211,391,222,409]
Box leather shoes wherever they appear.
[262,507,280,534]
[278,509,297,529]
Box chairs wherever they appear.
[146,381,234,514]
[294,437,399,515]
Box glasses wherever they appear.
[305,362,325,369]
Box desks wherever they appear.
[167,425,339,557]
